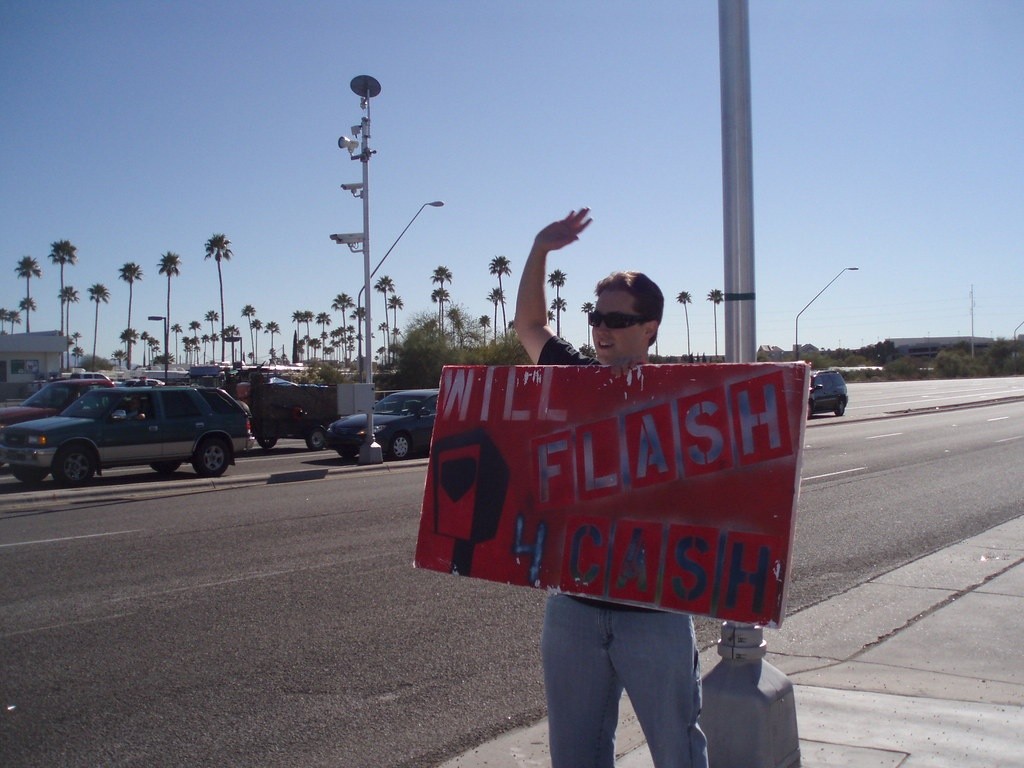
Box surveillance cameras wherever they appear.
[330,232,364,244]
[340,183,364,190]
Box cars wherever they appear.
[27,363,166,407]
[324,387,440,462]
[0,377,116,469]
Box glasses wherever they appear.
[588,312,653,329]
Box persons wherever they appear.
[127,396,147,419]
[515,207,710,768]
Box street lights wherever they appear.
[359,200,448,468]
[795,266,859,362]
[146,316,170,385]
[224,336,244,366]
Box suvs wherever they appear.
[0,385,253,488]
[807,370,848,422]
[252,383,326,452]
